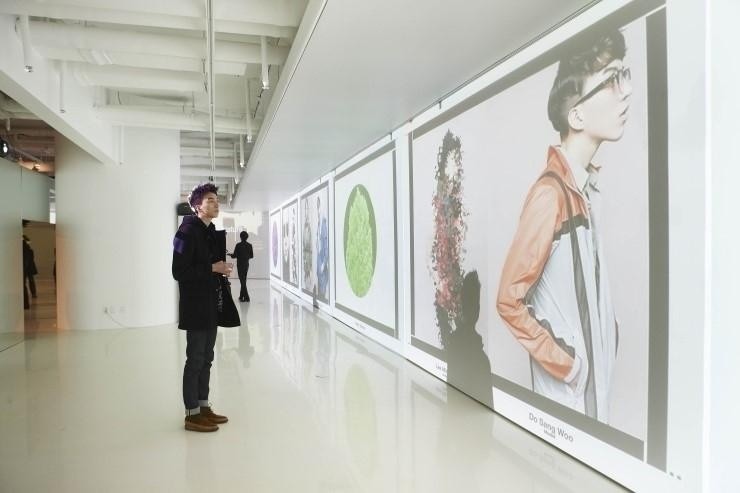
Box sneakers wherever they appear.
[238,295,250,301]
[185,414,219,432]
[200,406,228,423]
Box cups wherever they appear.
[223,262,234,277]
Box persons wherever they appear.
[281,196,328,299]
[23,234,31,308]
[172,182,241,433]
[226,230,254,302]
[495,29,634,427]
[26,238,38,298]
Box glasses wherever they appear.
[573,66,631,108]
